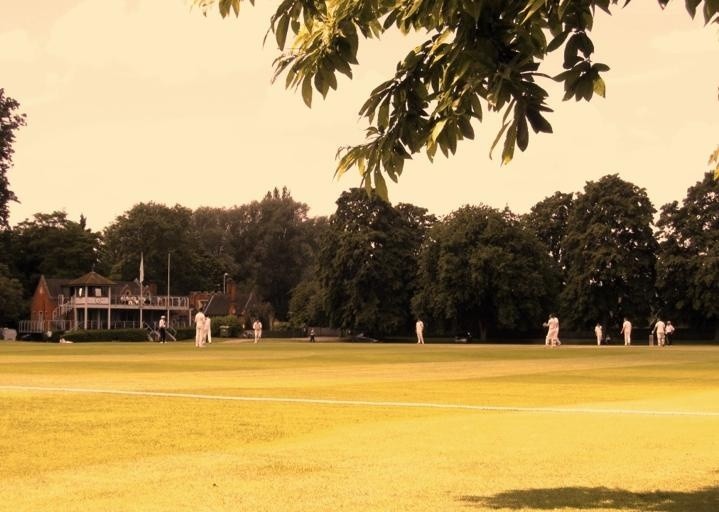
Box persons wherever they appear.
[542,313,561,347]
[310,328,316,343]
[620,318,633,345]
[594,322,603,347]
[415,318,424,345]
[158,315,167,344]
[651,317,675,347]
[194,308,212,348]
[252,319,263,345]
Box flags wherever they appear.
[139,255,146,284]
